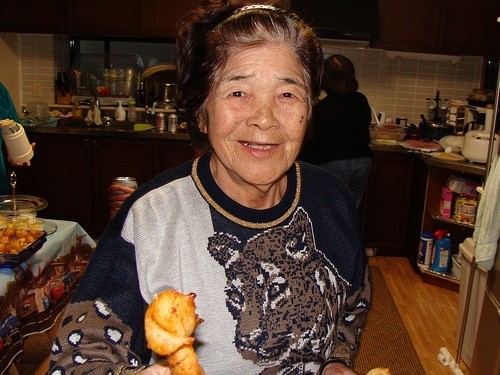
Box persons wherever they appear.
[43,0,370,375]
[300,55,372,248]
[0,81,36,197]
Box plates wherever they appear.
[0,215,58,238]
[0,194,47,214]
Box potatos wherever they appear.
[0,227,44,255]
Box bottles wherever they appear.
[432,233,450,272]
[102,65,125,95]
[115,101,137,122]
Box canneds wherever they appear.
[111,177,137,202]
[157,113,167,130]
[168,114,178,133]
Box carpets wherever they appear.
[350,265,425,375]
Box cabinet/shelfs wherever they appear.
[0,0,500,57]
[364,150,427,255]
[416,155,485,285]
[21,130,162,234]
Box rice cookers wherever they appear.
[462,130,500,164]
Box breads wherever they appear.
[365,368,391,375]
[145,290,203,375]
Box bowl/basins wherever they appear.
[420,120,455,141]
[465,98,489,107]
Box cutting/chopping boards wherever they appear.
[369,123,404,140]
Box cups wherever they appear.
[89,79,101,95]
[2,123,34,164]
